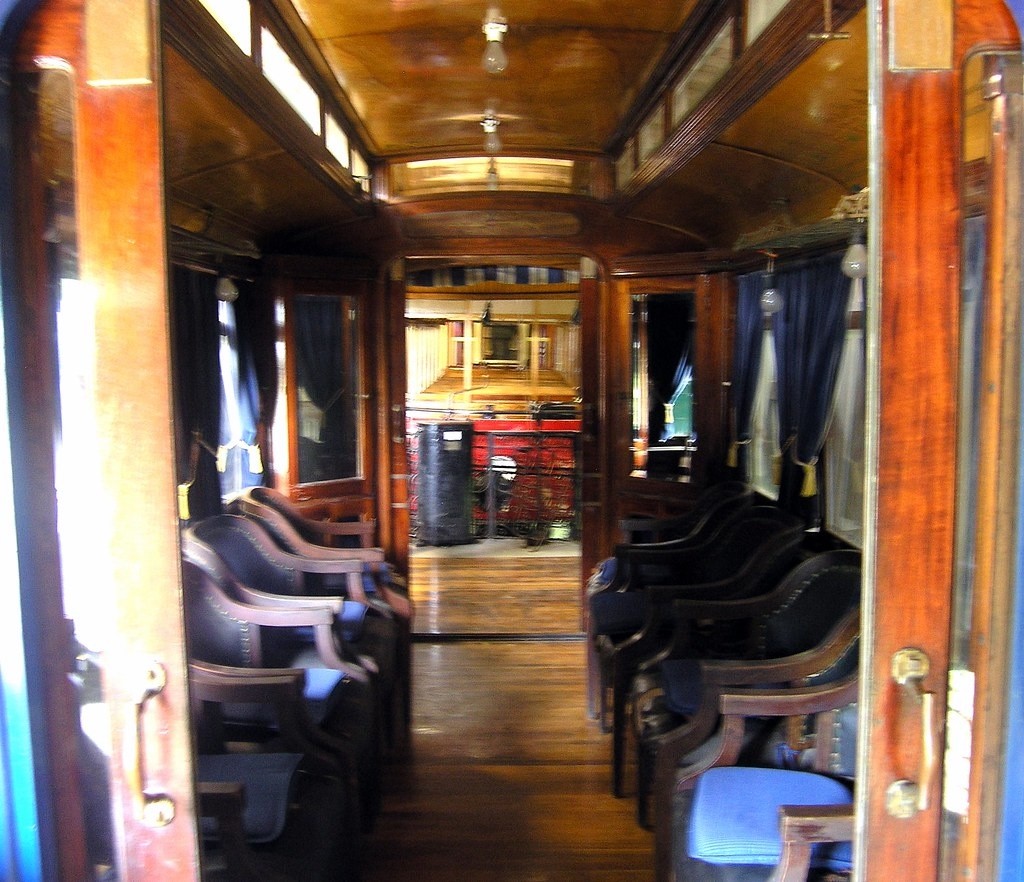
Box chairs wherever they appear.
[188,670,360,882]
[590,501,808,795]
[634,546,867,845]
[587,480,754,718]
[183,556,375,848]
[237,486,416,767]
[655,668,860,882]
[182,512,401,815]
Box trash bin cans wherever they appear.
[416,422,475,545]
